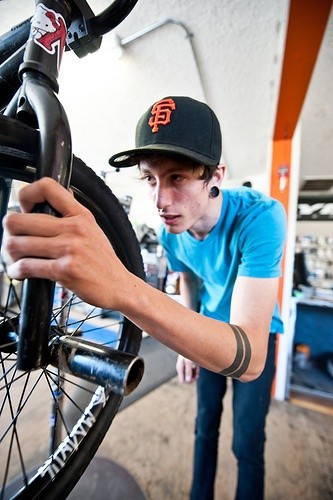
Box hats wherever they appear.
[108,99,222,167]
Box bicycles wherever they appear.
[0,0,153,500]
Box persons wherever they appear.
[0,94,287,500]
